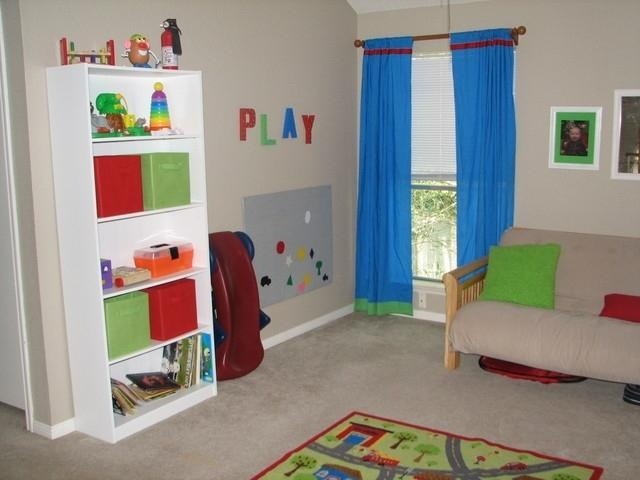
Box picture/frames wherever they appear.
[611,89,640,179]
[548,107,602,170]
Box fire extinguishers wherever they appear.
[160,19,182,70]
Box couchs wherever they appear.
[443,227,640,385]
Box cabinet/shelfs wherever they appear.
[47,63,217,444]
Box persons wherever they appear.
[563,128,588,156]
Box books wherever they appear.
[110,333,213,416]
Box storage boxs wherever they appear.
[134,240,195,277]
